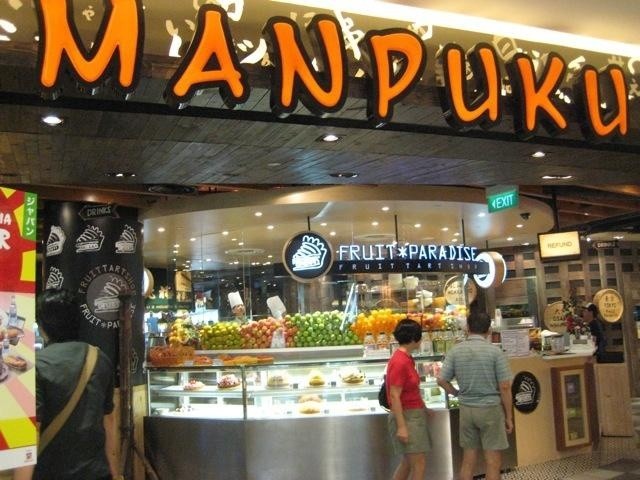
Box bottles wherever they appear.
[8,294,18,328]
[363,328,466,358]
[574,324,581,339]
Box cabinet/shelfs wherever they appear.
[145,355,459,421]
[564,373,586,441]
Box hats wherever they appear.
[581,302,596,312]
[266,296,286,317]
[227,290,243,308]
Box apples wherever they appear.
[199,310,363,350]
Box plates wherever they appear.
[3,353,35,375]
[0,364,9,381]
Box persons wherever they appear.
[580,302,608,361]
[266,295,289,348]
[227,290,258,323]
[4,299,117,480]
[382,319,431,480]
[435,310,516,480]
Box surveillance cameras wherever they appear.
[520,213,530,219]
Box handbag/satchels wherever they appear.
[378,384,389,409]
